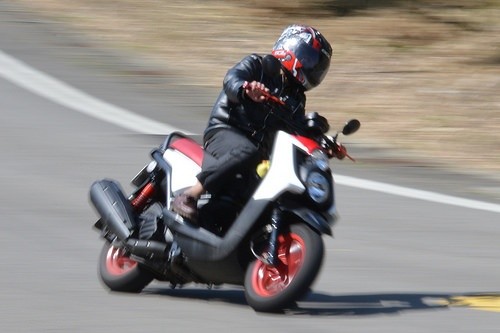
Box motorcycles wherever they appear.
[89,80,361,312]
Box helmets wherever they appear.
[273,25,334,89]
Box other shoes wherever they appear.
[172,192,200,222]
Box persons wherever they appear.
[171,22,347,229]
